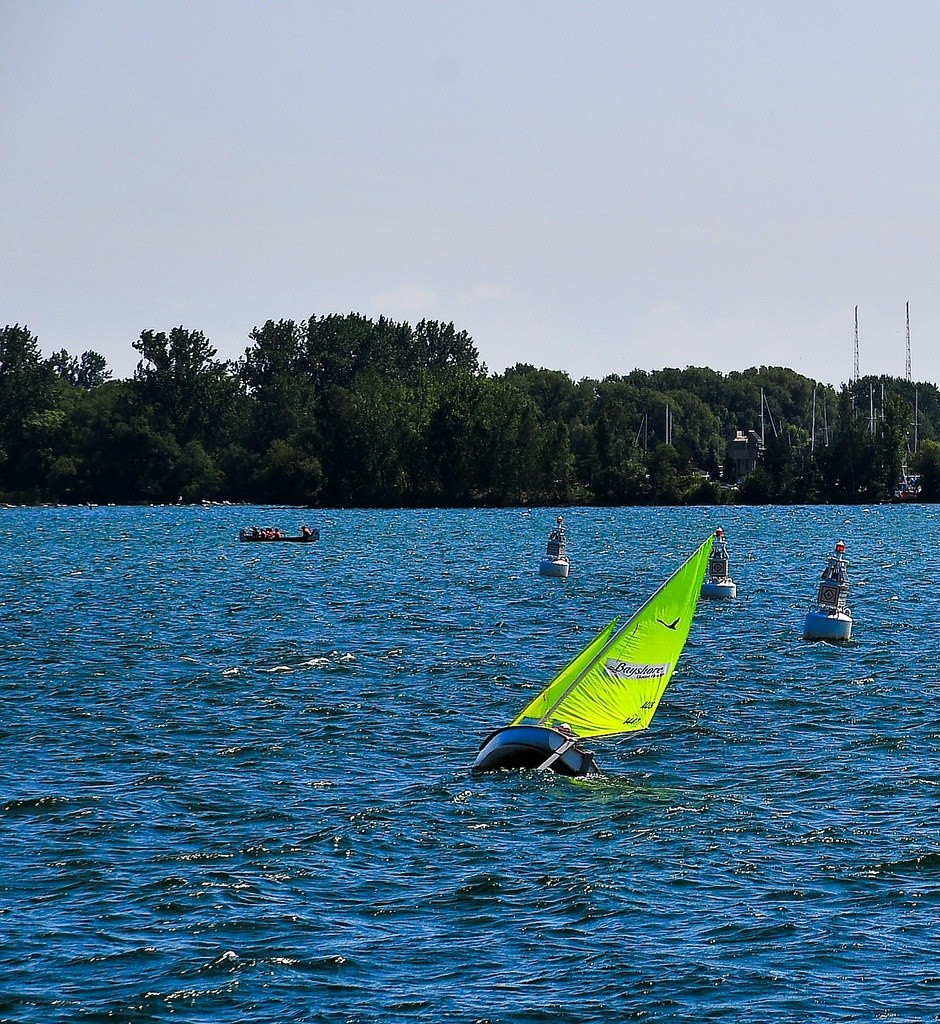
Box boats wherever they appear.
[240,527,322,542]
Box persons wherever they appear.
[302,525,313,538]
[552,723,572,737]
[251,526,282,539]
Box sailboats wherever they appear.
[468,525,723,779]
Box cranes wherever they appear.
[904,301,915,384]
[854,304,861,385]
